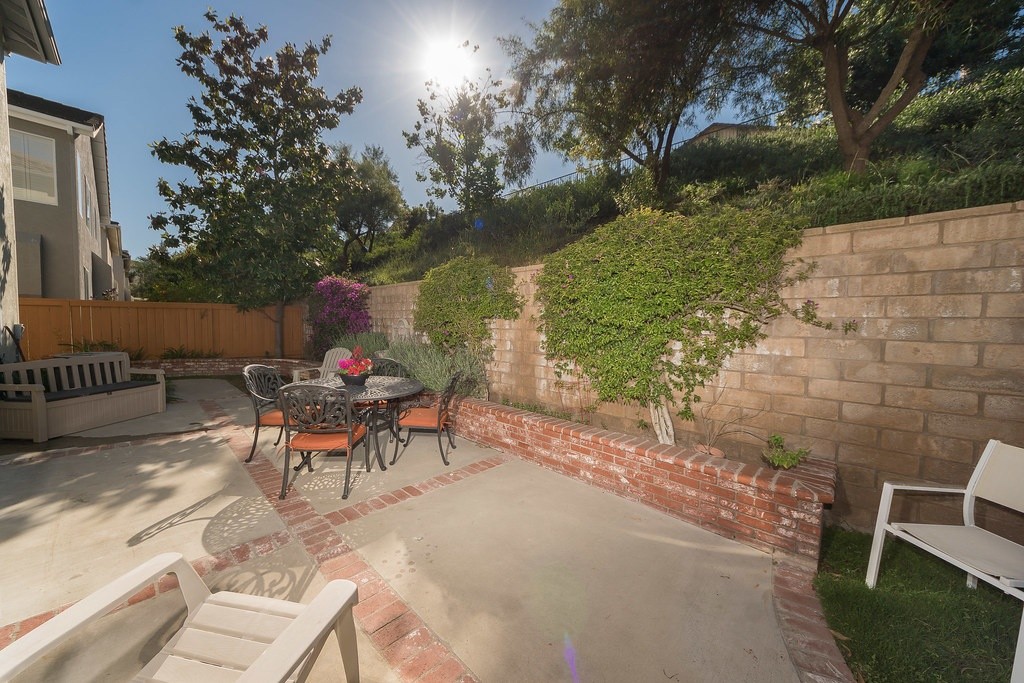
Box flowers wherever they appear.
[334,357,376,376]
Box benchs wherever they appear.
[865,438,1024,683]
[0,352,167,444]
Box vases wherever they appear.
[338,373,371,387]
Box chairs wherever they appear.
[277,382,371,500]
[242,362,317,465]
[387,369,462,467]
[0,552,360,683]
[319,347,404,445]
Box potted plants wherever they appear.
[690,382,814,468]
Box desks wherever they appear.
[277,375,424,471]
